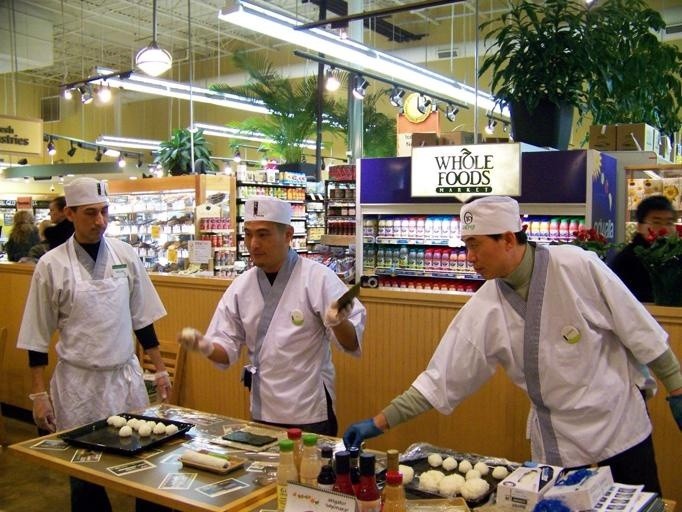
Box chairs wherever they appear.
[138,338,187,405]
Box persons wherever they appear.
[344,195,682,496]
[16,176,171,512]
[17,220,55,264]
[178,194,368,437]
[40,197,75,250]
[609,194,674,303]
[7,211,39,261]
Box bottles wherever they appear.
[275,426,407,512]
[197,168,307,276]
[523,218,587,246]
[362,217,482,292]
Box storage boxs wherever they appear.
[590,122,671,162]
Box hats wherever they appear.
[64,177,111,207]
[244,196,293,226]
[459,194,523,237]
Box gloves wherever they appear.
[342,418,385,451]
[665,393,682,433]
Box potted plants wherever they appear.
[471,0,650,152]
